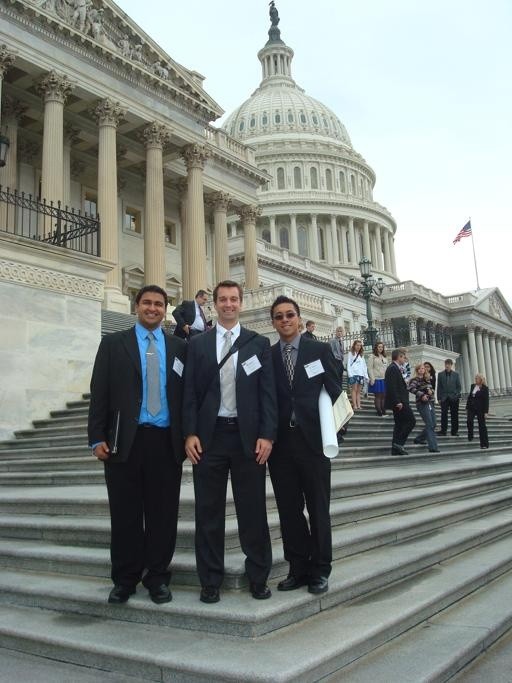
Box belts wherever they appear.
[286,420,298,430]
[217,416,238,425]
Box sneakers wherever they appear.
[376,411,387,416]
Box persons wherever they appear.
[346,326,441,456]
[182,281,278,604]
[436,359,461,436]
[172,288,210,341]
[265,294,349,595]
[466,373,489,449]
[303,320,315,339]
[330,326,344,363]
[86,284,188,605]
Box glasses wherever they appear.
[273,313,297,321]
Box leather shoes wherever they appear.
[391,432,489,455]
[277,576,306,591]
[200,585,220,603]
[148,583,172,604]
[308,575,328,594]
[108,584,136,604]
[249,583,271,600]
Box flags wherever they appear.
[453,221,472,244]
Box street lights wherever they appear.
[346,255,385,348]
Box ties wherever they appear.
[221,332,236,412]
[284,345,296,392]
[199,307,207,331]
[147,334,161,418]
[447,373,449,383]
